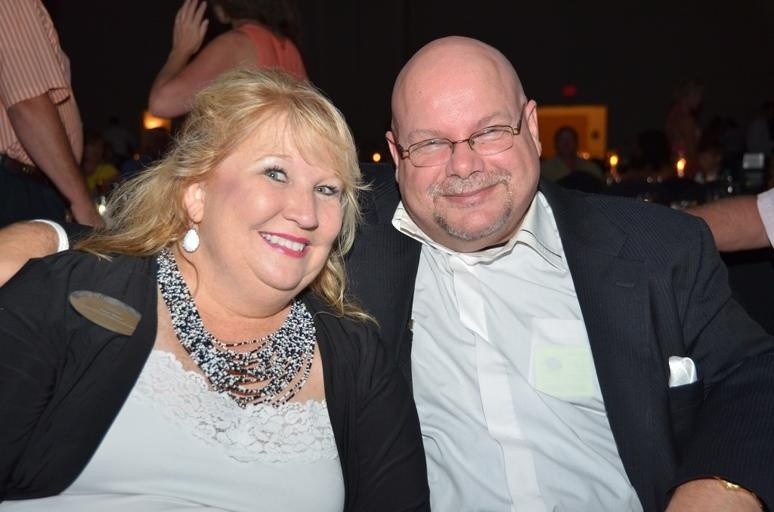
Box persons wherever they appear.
[0,60,431,510]
[536,63,772,257]
[119,127,173,181]
[0,34,773,512]
[148,1,312,121]
[0,1,104,234]
[79,133,119,198]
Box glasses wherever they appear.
[392,107,528,167]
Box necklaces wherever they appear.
[153,248,317,409]
